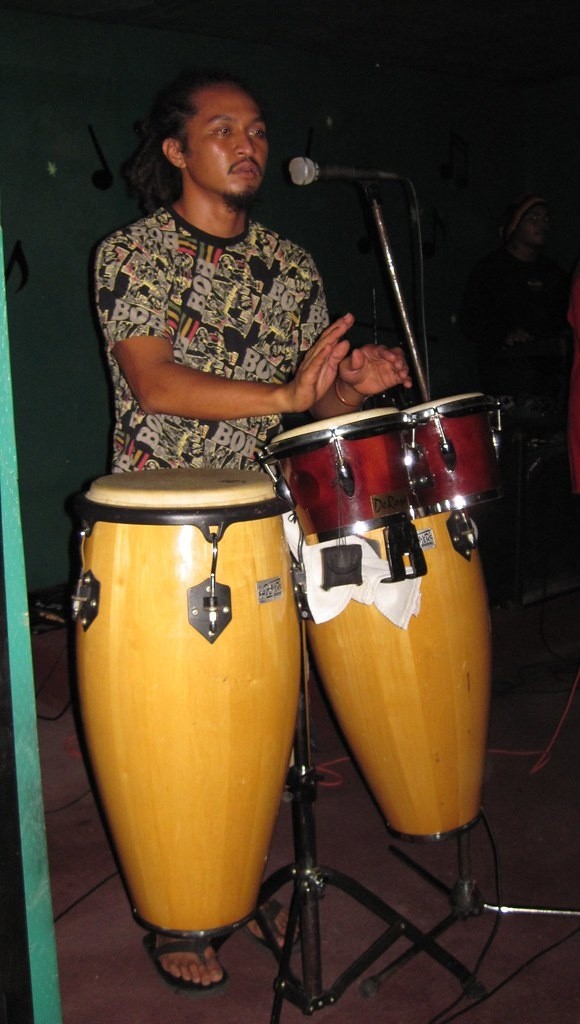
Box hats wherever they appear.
[501,191,549,240]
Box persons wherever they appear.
[94,71,414,998]
[460,189,571,610]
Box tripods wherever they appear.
[208,682,579,1024]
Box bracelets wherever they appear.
[335,381,362,406]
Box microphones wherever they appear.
[288,156,408,185]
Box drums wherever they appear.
[71,468,302,937]
[403,392,513,518]
[271,408,411,545]
[270,511,492,843]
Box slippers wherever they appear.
[239,899,302,953]
[142,930,229,993]
